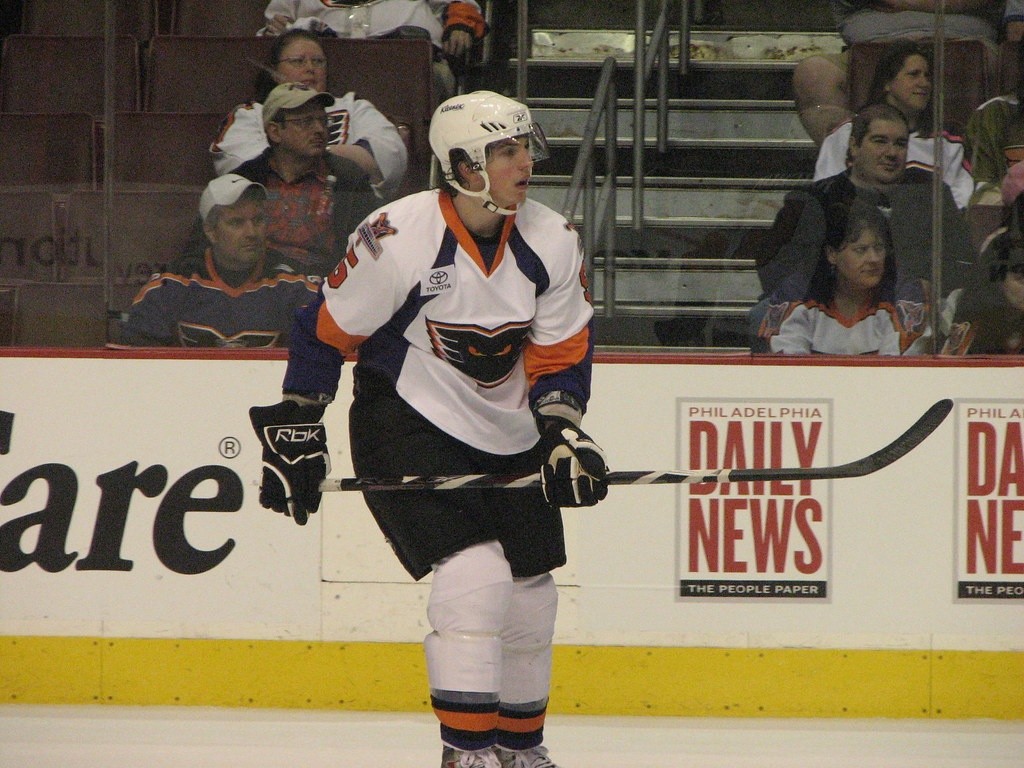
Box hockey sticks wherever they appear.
[315,396,953,493]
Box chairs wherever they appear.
[0,0,435,349]
[849,37,1024,135]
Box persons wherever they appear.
[757,1,1024,359]
[210,30,408,200]
[249,89,609,768]
[183,80,381,275]
[255,0,493,98]
[108,172,326,347]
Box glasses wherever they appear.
[281,117,329,129]
[279,57,328,68]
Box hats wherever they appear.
[263,82,335,130]
[199,174,267,220]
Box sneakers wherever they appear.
[441,746,501,768]
[492,745,559,768]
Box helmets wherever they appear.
[429,91,550,174]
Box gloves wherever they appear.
[249,400,331,526]
[535,423,608,507]
